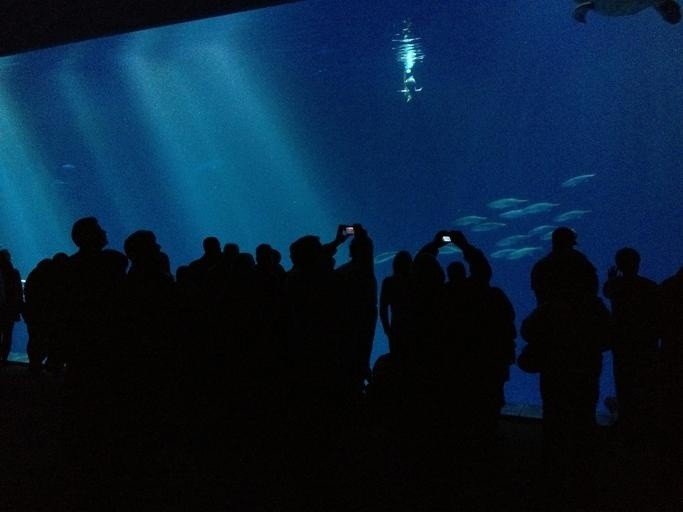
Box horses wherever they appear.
[441,235,452,242]
[344,226,354,234]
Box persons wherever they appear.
[3,249,23,366]
[62,217,378,463]
[603,248,679,467]
[516,227,610,468]
[369,231,516,459]
[23,253,70,377]
